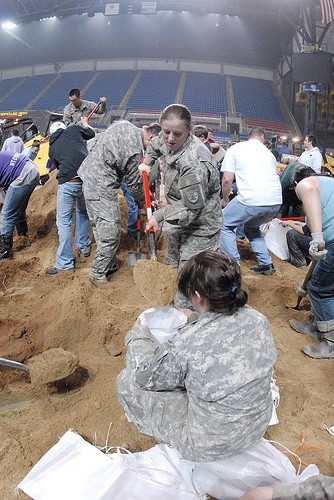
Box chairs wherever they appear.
[0,70,293,145]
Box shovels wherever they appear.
[87,101,101,122]
[128,205,148,269]
[0,348,79,386]
[287,242,326,311]
[133,149,179,307]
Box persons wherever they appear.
[0,150,40,259]
[139,104,225,313]
[207,128,215,143]
[238,475,334,500]
[21,140,40,160]
[1,128,25,153]
[218,127,284,276]
[193,125,211,154]
[298,134,323,173]
[116,251,278,461]
[76,120,165,283]
[62,89,106,124]
[290,165,334,360]
[264,137,292,161]
[45,117,95,275]
[279,220,314,268]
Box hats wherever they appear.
[210,143,226,162]
[50,122,66,134]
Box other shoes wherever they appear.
[0,222,333,358]
